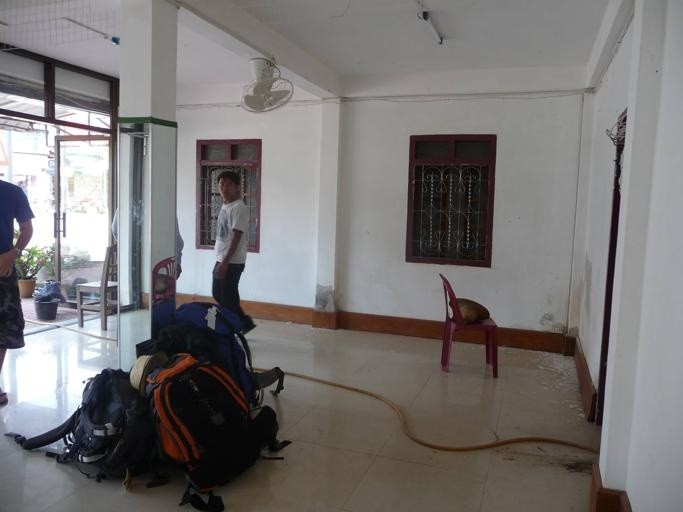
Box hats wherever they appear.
[449,299,489,324]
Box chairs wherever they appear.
[152,256,176,301]
[439,273,498,379]
[75,243,117,332]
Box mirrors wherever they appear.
[151,117,177,339]
[118,117,151,371]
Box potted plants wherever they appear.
[65,278,90,309]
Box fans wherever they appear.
[240,57,294,114]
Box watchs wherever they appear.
[12,246,23,258]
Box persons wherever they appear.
[209,170,257,340]
[0,180,35,408]
[111,205,184,280]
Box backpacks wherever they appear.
[5,369,153,483]
[125,301,284,512]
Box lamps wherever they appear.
[422,11,443,45]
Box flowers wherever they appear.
[14,246,48,279]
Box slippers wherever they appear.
[0,393,8,405]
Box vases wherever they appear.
[17,280,37,299]
[34,300,57,320]
